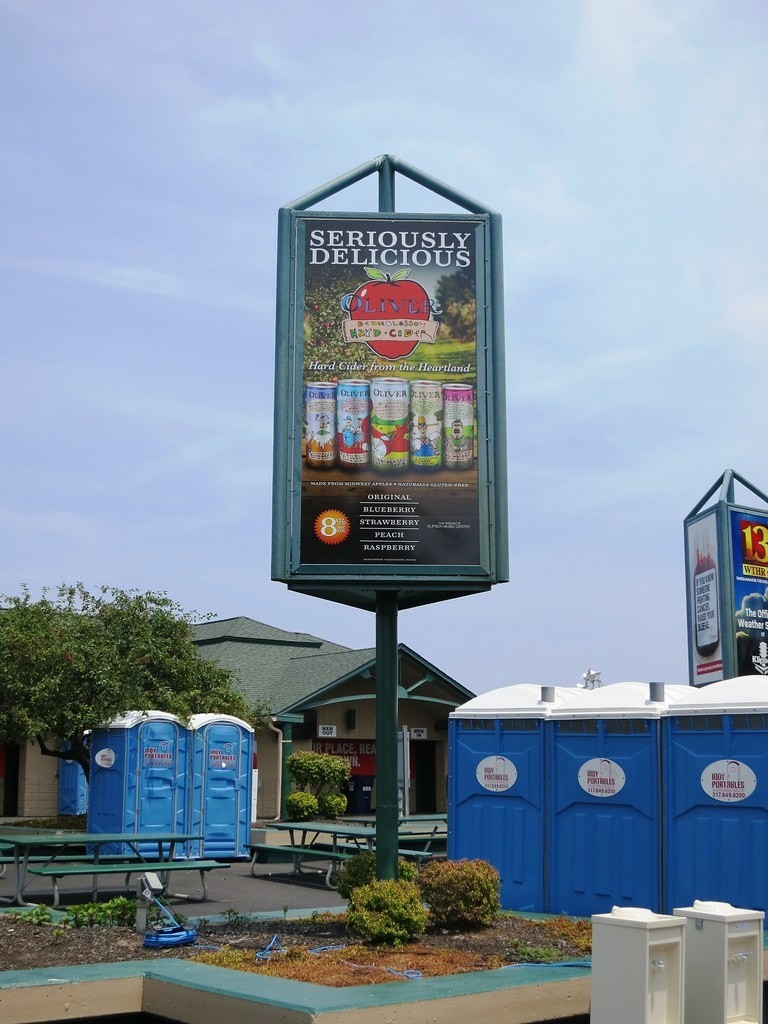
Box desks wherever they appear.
[0,842,15,877]
[267,814,448,890]
[0,834,204,910]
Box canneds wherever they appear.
[304,377,473,474]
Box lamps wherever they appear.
[348,709,356,730]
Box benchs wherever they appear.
[242,831,448,890]
[0,855,231,910]
